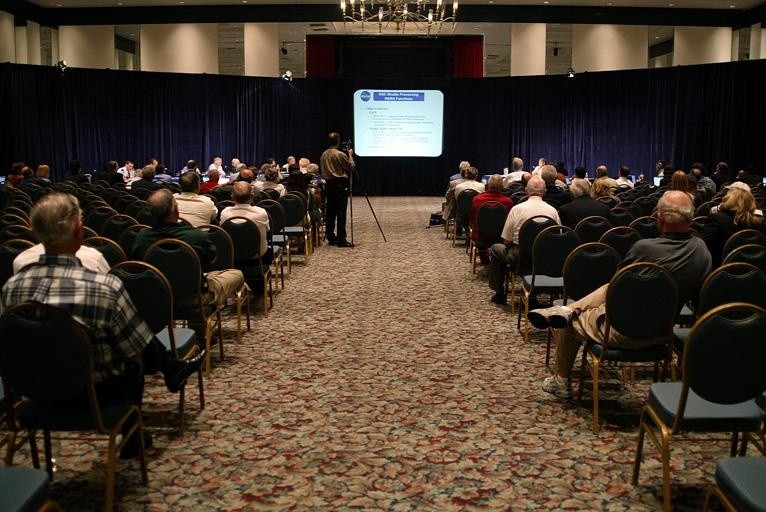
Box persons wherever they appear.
[1,153,316,454]
[319,132,356,247]
[448,157,766,398]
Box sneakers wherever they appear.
[528,305,573,329]
[542,375,573,398]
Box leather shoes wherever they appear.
[337,241,353,247]
[165,350,206,393]
[119,431,152,459]
[328,238,336,245]
[492,294,506,304]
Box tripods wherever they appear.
[344,166,386,247]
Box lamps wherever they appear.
[341,0,458,39]
[283,69,293,80]
[567,67,575,77]
[57,60,67,68]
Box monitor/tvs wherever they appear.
[565,176,571,185]
[762,176,766,187]
[202,175,208,182]
[0,175,5,184]
[628,174,636,182]
[653,176,663,187]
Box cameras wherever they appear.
[339,139,351,151]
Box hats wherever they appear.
[725,181,752,193]
